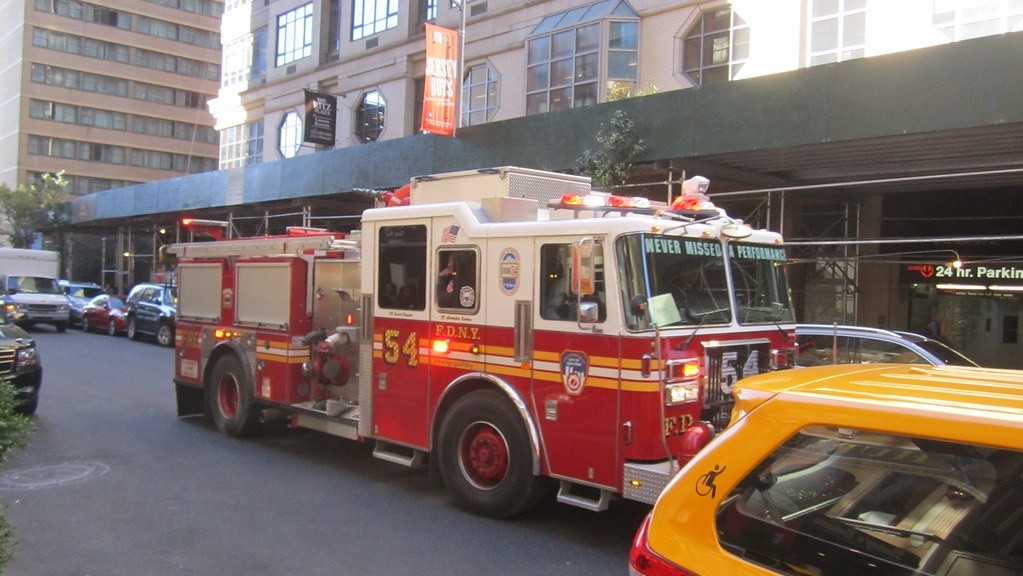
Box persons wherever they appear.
[926,320,952,360]
[438,254,475,308]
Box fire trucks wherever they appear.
[168,168,801,522]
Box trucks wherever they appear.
[0,248,68,331]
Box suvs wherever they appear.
[124,280,174,346]
[59,280,103,327]
[800,324,973,365]
[0,308,43,416]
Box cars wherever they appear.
[83,295,124,337]
[630,365,1023,576]
